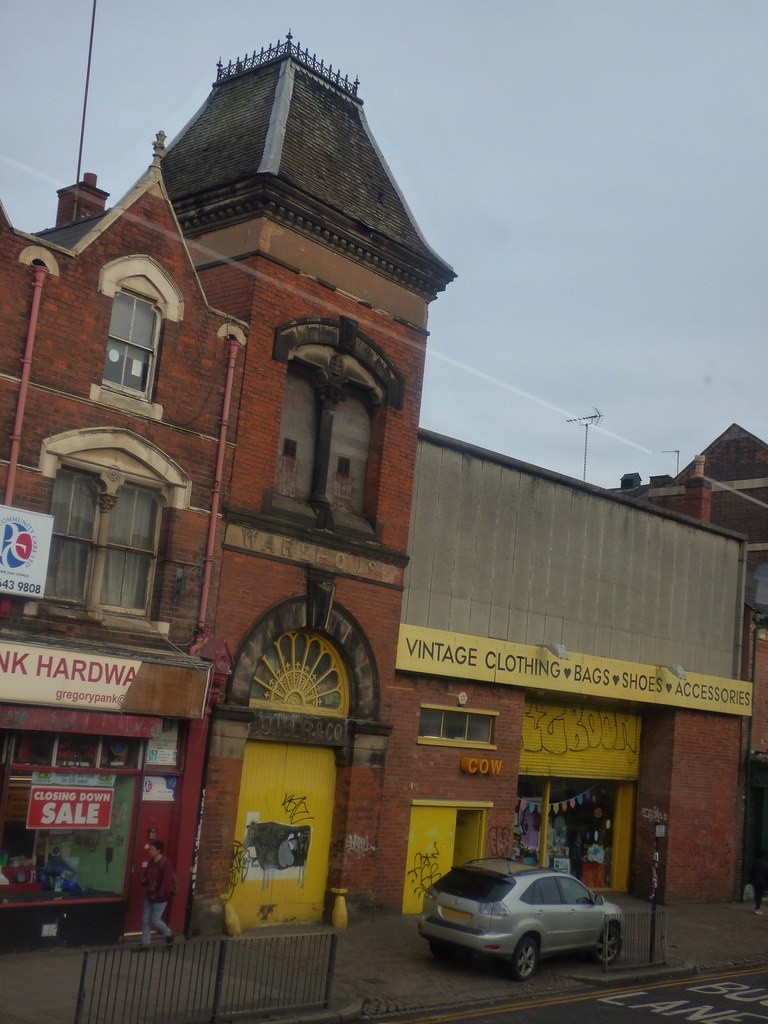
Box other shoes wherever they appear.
[754,909,763,914]
[131,943,151,952]
[166,931,174,950]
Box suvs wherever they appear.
[417,857,624,981]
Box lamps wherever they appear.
[657,664,687,681]
[534,641,571,661]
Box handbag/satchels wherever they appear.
[156,878,176,897]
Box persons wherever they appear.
[130,840,179,951]
